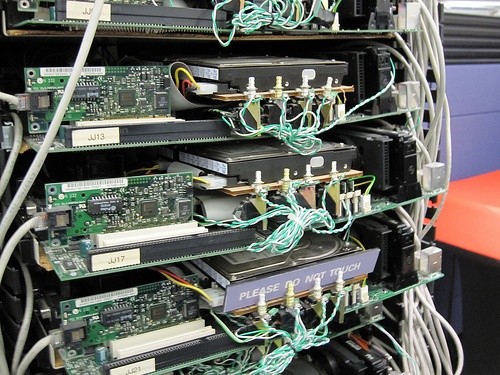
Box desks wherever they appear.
[423,165,500,375]
